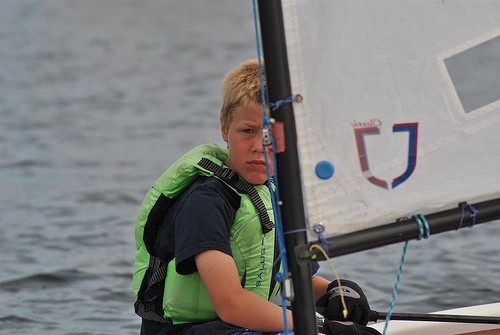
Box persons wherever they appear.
[134,60,383,335]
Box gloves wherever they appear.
[316,279,370,326]
[322,320,381,335]
